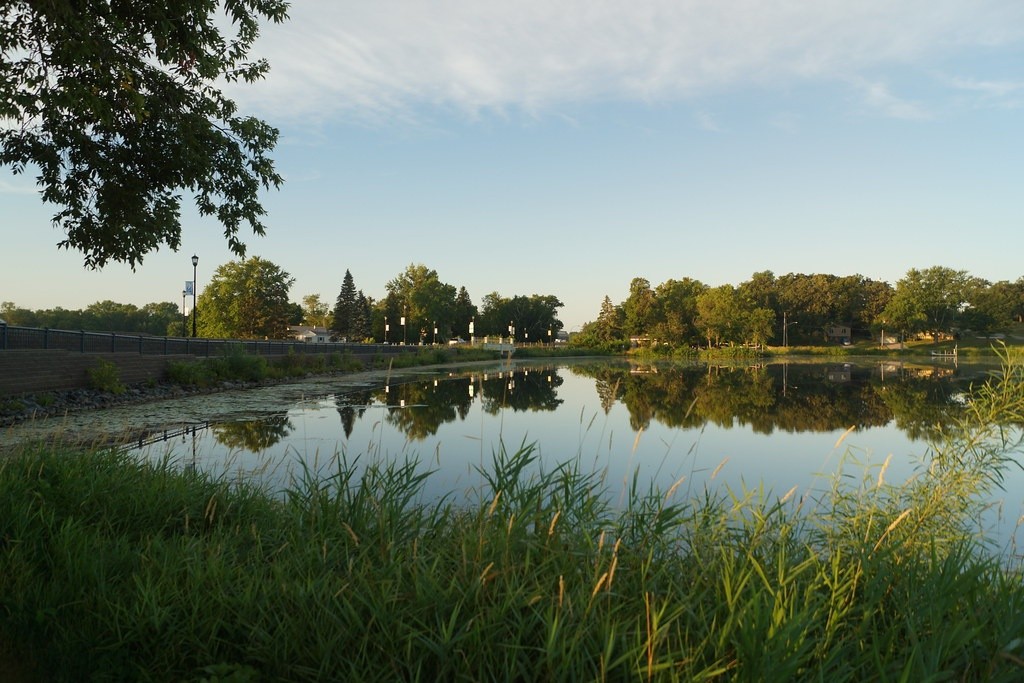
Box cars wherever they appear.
[841,337,850,346]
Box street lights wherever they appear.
[511,320,514,344]
[383,316,389,344]
[190,253,199,336]
[550,323,552,346]
[471,315,475,346]
[403,304,407,346]
[432,320,436,346]
[786,321,797,346]
[182,290,187,337]
[524,327,527,347]
